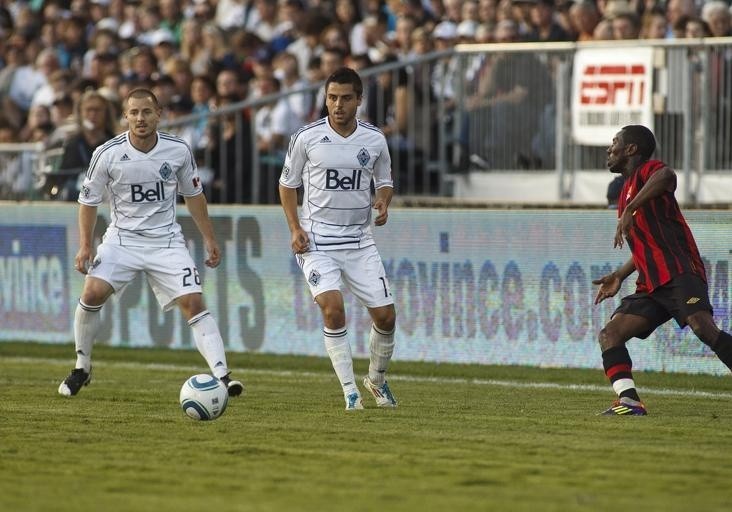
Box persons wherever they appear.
[58,90,244,397]
[591,126,732,414]
[278,68,401,410]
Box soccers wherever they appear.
[179,374,229,421]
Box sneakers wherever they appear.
[343,389,365,411]
[220,370,244,397]
[57,361,95,398]
[362,373,399,410]
[593,397,649,420]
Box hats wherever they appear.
[95,16,176,47]
[456,18,476,39]
[432,20,459,40]
